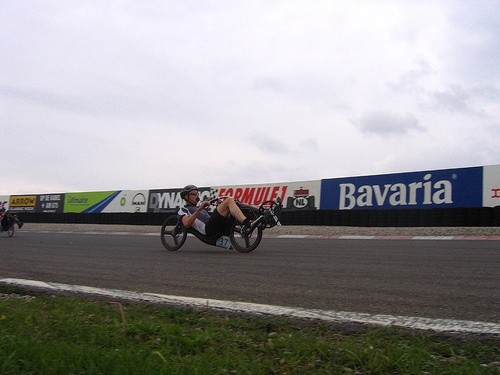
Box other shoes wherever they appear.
[19,222,23,229]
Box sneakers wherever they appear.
[241,215,263,234]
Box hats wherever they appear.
[180,185,198,199]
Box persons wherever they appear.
[0,201,24,229]
[178,185,282,236]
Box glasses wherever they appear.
[187,190,198,195]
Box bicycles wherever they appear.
[160,196,281,254]
[0,201,24,237]
